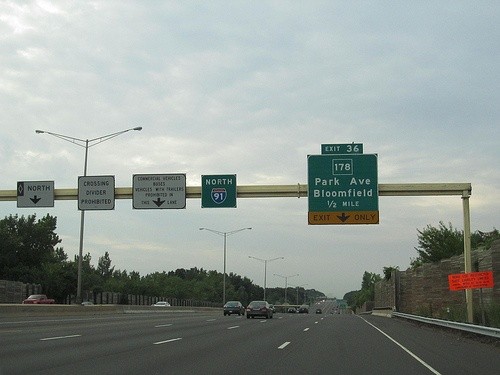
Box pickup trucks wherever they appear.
[21,294,55,304]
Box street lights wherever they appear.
[273,272,300,305]
[199,226,252,308]
[35,126,143,306]
[248,255,284,302]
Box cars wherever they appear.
[153,301,171,307]
[223,300,245,316]
[299,305,309,313]
[287,306,297,313]
[245,301,273,319]
[316,308,322,314]
[268,303,276,313]
[81,302,93,306]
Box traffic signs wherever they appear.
[132,173,187,210]
[306,143,380,225]
[17,181,54,208]
